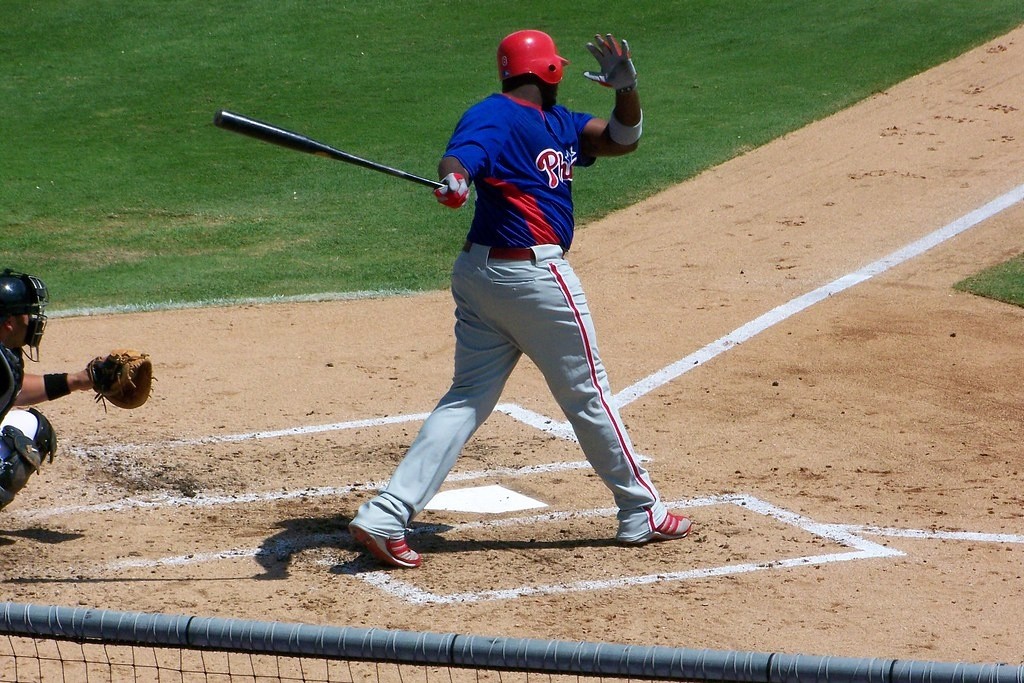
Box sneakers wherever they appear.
[350,521,421,568]
[632,513,691,544]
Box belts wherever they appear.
[463,241,536,261]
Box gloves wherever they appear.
[432,173,470,209]
[583,34,638,91]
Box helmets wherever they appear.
[0,271,46,315]
[497,30,569,84]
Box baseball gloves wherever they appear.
[86,348,158,414]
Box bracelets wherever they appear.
[44,373,71,401]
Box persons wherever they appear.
[348,30,692,569]
[0,269,152,512]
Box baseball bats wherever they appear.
[213,109,452,191]
